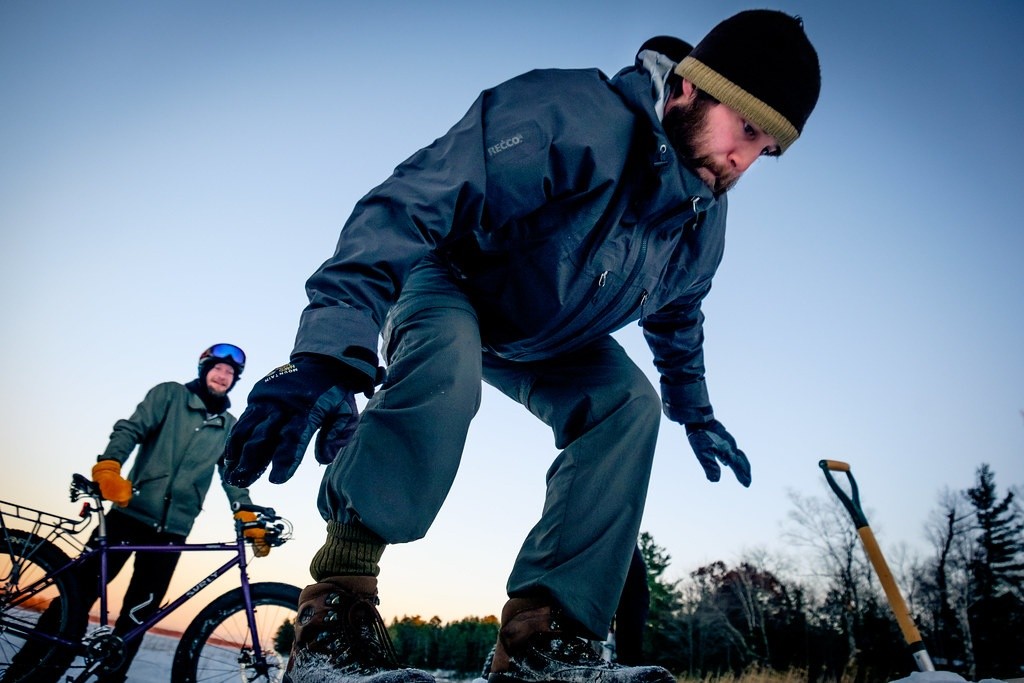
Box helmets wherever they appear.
[198,343,245,394]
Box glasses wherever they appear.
[200,343,246,371]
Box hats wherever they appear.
[674,8,822,156]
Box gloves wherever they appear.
[92,459,132,507]
[223,354,361,486]
[234,512,271,558]
[684,419,752,488]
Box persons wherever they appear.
[223,10,821,683]
[614,547,650,669]
[0,343,271,683]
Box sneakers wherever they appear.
[487,596,678,683]
[283,574,436,683]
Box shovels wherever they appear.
[818,458,934,671]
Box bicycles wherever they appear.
[0,471,310,683]
